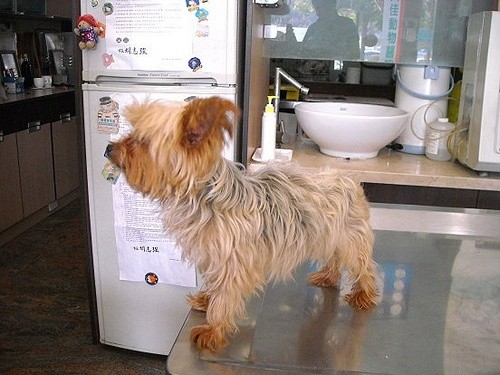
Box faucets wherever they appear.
[273,66,310,148]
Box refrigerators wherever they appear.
[80,0,248,356]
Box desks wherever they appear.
[165,201,500,375]
[240,148,500,194]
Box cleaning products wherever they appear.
[260,95,280,160]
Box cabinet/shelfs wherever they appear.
[0,112,82,248]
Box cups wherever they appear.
[344,67,360,84]
[2,77,24,95]
[34,77,46,89]
[42,75,53,89]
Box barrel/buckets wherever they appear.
[361,63,395,87]
[391,64,454,155]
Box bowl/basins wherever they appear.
[294,102,411,159]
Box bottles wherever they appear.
[427,117,456,161]
[20,54,35,90]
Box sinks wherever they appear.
[294,101,411,161]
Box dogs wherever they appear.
[103,96,381,350]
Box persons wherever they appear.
[302,0,360,60]
